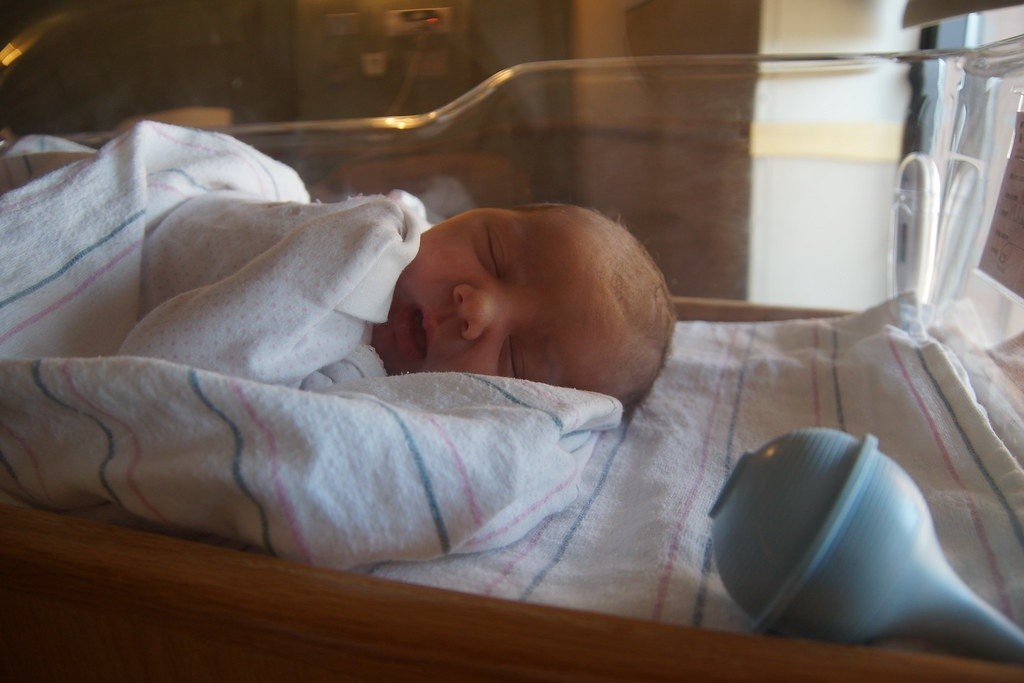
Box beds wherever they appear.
[0,32,1024,683]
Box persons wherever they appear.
[0,134,676,414]
